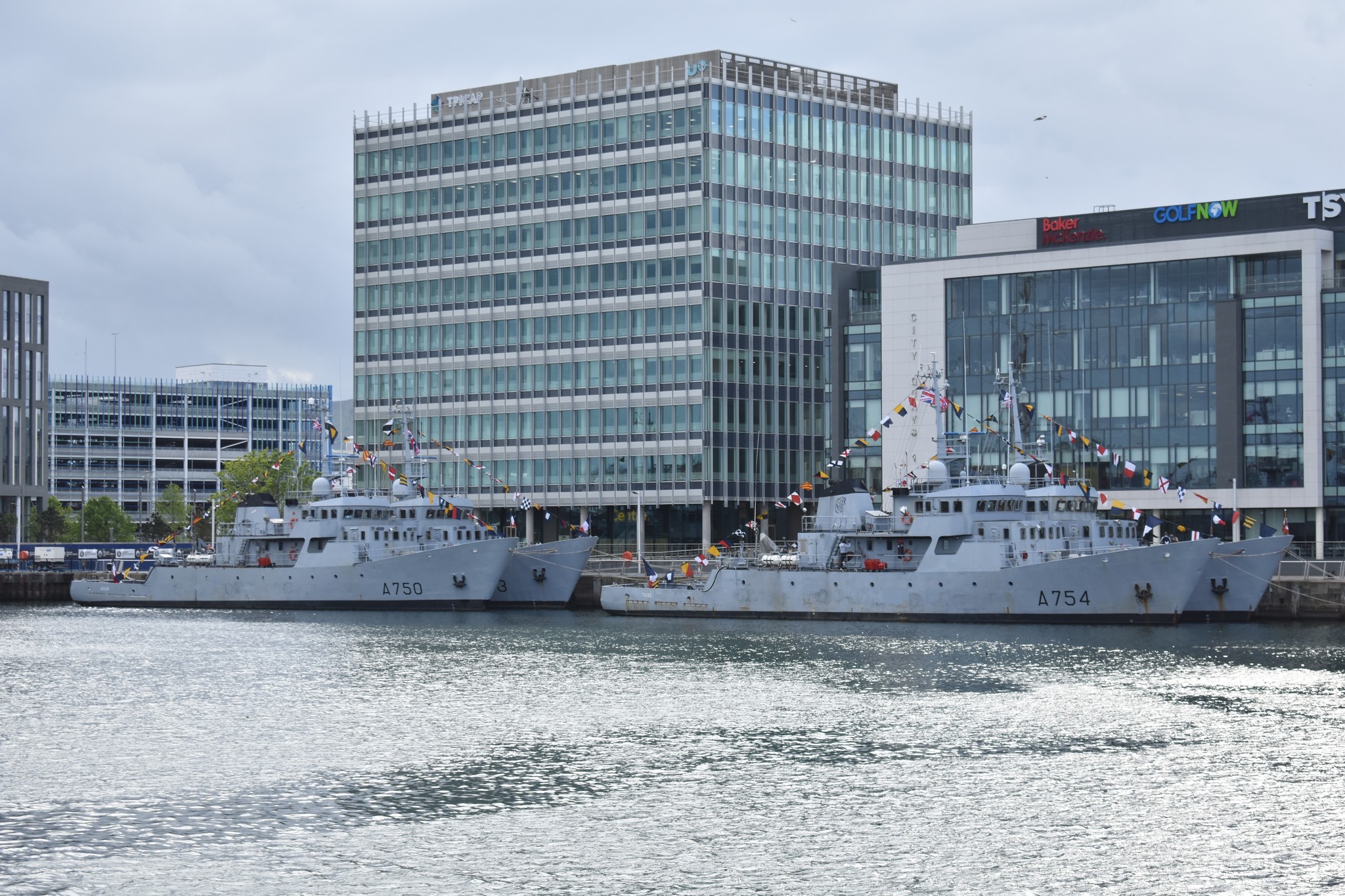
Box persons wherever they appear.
[839,540,852,572]
[1170,534,1179,542]
[1037,435,1045,458]
[1159,532,1171,545]
[206,544,214,555]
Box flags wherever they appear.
[641,378,935,589]
[111,418,591,584]
[940,389,1288,543]
[579,516,590,532]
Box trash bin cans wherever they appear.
[20,561,27,570]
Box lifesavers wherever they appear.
[902,549,912,562]
[902,513,912,526]
[289,550,296,560]
[289,518,297,528]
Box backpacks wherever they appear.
[1163,536,1170,544]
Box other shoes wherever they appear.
[840,568,844,572]
[842,561,845,566]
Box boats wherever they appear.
[70,399,600,611]
[600,311,1295,626]
